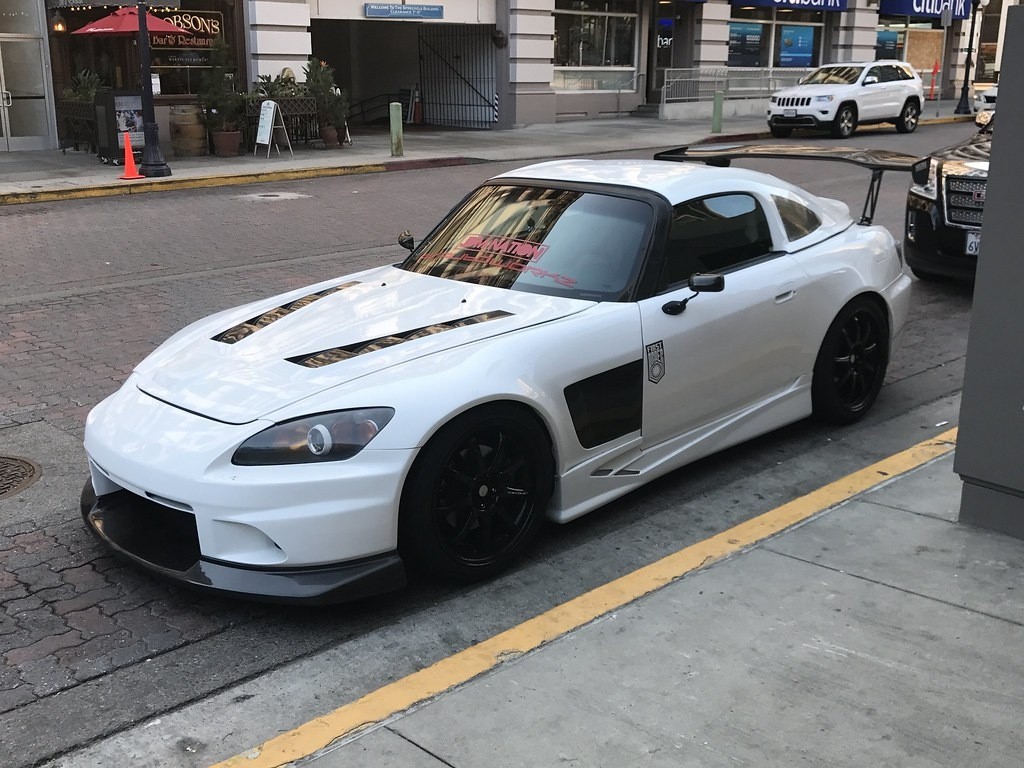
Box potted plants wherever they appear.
[302,59,350,147]
[191,57,243,153]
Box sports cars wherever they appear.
[80,141,931,614]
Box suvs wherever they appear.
[765,57,925,139]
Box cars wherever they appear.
[902,112,994,281]
[973,81,998,112]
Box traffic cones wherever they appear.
[118,134,147,181]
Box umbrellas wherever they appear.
[68,5,196,93]
